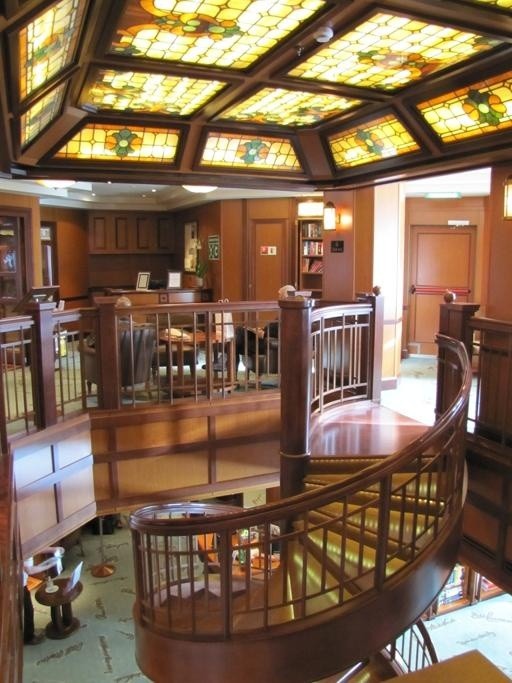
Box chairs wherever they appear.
[78,326,155,401]
[242,308,316,392]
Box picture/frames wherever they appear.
[182,220,200,273]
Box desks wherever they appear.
[35,578,82,640]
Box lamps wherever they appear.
[501,173,512,220]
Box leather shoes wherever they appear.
[202,353,227,370]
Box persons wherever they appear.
[23,546,65,645]
[87,296,156,348]
[202,285,297,371]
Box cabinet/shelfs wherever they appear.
[299,219,324,301]
[422,562,506,622]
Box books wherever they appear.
[302,223,324,273]
